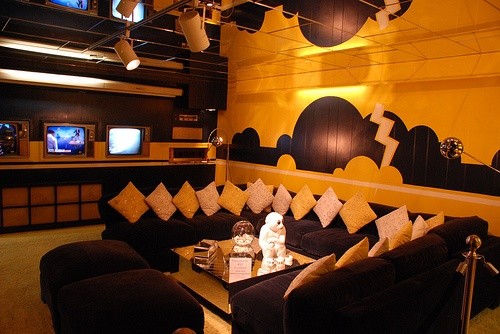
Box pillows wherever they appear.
[171,181,198,219]
[369,237,390,258]
[290,185,317,219]
[313,187,343,227]
[376,204,409,240]
[194,181,221,216]
[244,178,274,214]
[271,184,293,215]
[108,181,149,222]
[218,180,248,215]
[389,220,413,249]
[145,182,176,221]
[411,210,445,241]
[284,253,339,300]
[339,192,378,232]
[336,237,372,271]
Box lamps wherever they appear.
[114,0,210,71]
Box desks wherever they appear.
[171,235,318,320]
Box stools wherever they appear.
[40,239,204,334]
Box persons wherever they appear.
[47,130,58,151]
[74,128,80,141]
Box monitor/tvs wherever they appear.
[104,125,150,157]
[108,0,147,24]
[42,123,96,158]
[0,120,30,157]
[43,0,99,18]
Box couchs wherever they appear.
[99,183,489,334]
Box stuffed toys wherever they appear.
[259,212,293,261]
